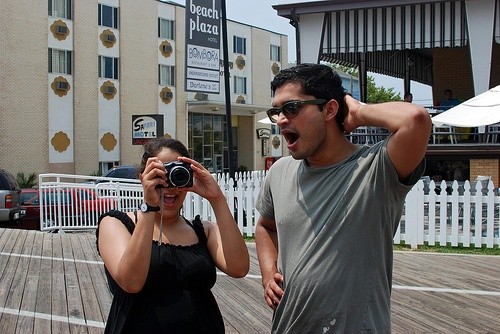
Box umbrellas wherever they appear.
[430,85,500,127]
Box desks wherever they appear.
[425,105,453,111]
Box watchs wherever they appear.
[140,203,160,213]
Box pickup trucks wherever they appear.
[0,189,12,222]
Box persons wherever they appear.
[95,137,250,334]
[440,89,461,142]
[404,93,413,102]
[255,63,432,334]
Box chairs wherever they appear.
[486,123,500,144]
[433,124,458,145]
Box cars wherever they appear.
[9,188,116,230]
[80,166,142,185]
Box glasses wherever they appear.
[266,99,326,123]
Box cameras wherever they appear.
[156,160,194,188]
